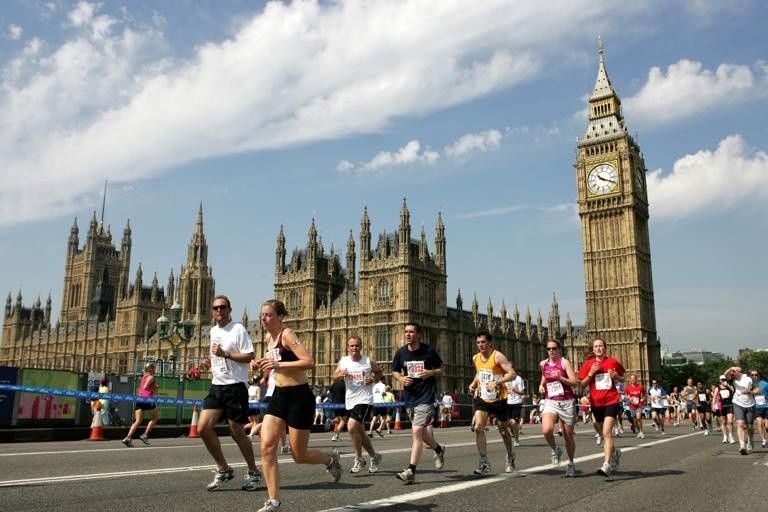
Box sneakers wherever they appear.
[558,430,563,436]
[612,423,664,439]
[122,437,134,447]
[282,446,291,453]
[505,453,515,473]
[510,432,520,447]
[474,462,491,474]
[594,433,602,445]
[721,433,768,455]
[257,500,282,512]
[597,449,621,476]
[139,434,149,444]
[435,446,445,468]
[367,428,392,439]
[242,469,263,491]
[551,447,575,477]
[206,466,235,490]
[368,454,381,472]
[351,458,365,473]
[328,450,343,483]
[331,436,339,440]
[247,434,254,442]
[673,422,720,437]
[395,468,415,484]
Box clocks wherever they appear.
[630,153,648,207]
[582,151,623,202]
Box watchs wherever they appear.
[222,351,230,359]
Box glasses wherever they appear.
[212,305,226,310]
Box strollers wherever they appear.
[109,400,133,426]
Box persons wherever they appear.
[98,380,113,425]
[334,335,382,473]
[315,369,768,446]
[725,367,755,453]
[469,331,516,473]
[243,367,292,455]
[122,362,159,447]
[390,323,446,482]
[538,339,576,477]
[249,299,342,512]
[577,338,625,475]
[197,295,263,492]
[90,385,102,430]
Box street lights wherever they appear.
[88,404,107,441]
[664,409,671,426]
[157,298,196,377]
[687,412,693,425]
[528,409,534,424]
[187,405,200,438]
[569,326,578,370]
[393,407,404,430]
[637,341,646,388]
[333,422,342,432]
[439,411,447,428]
